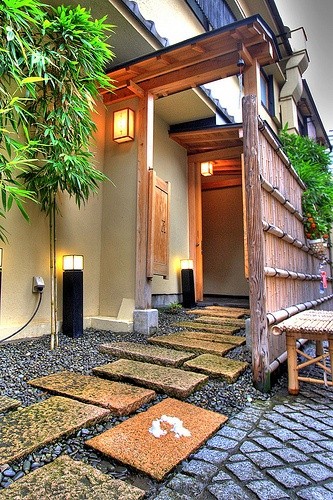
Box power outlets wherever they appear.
[32,276,46,293]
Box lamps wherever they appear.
[201,159,217,177]
[180,258,193,270]
[62,254,84,271]
[112,107,136,144]
[0,248,3,268]
